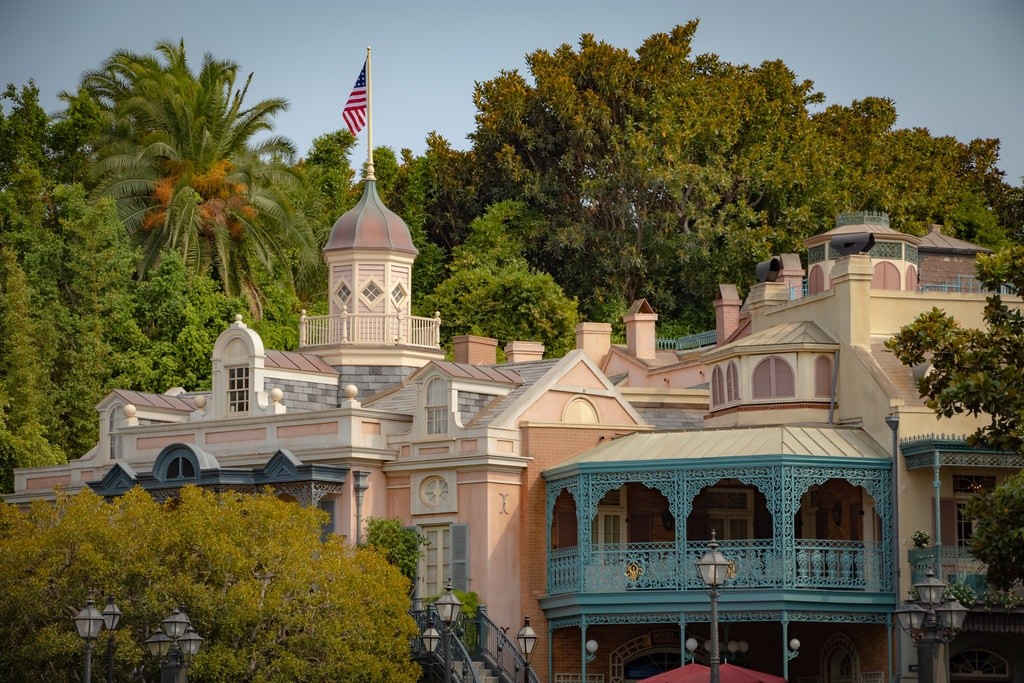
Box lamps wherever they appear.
[685,638,698,661]
[586,640,599,664]
[706,641,750,660]
[788,639,800,661]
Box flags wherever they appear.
[342,60,367,137]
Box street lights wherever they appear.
[695,528,738,683]
[892,567,970,683]
[515,614,540,683]
[418,575,465,683]
[145,603,204,683]
[71,591,122,683]
